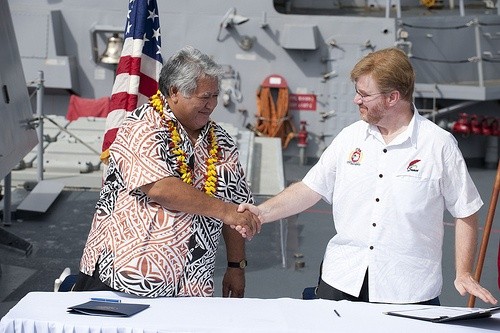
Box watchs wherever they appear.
[227,260,250,269]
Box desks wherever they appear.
[242,132,288,271]
[0,291,500,333]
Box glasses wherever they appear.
[355,85,386,101]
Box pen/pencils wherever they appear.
[331,305,341,317]
[89,297,121,302]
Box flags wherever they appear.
[100,0,165,168]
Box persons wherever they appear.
[228,49,499,306]
[74,47,262,301]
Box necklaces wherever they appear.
[148,93,218,198]
